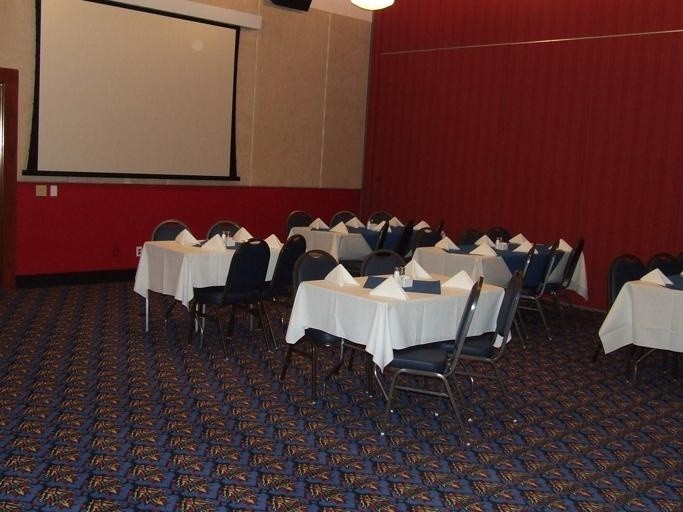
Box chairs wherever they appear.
[518,240,560,340]
[280,250,341,401]
[332,211,355,227]
[512,241,538,349]
[442,270,526,395]
[487,225,513,243]
[206,220,242,239]
[285,209,313,238]
[375,217,390,250]
[396,219,414,257]
[360,249,407,278]
[544,235,586,309]
[411,227,444,257]
[368,211,394,227]
[259,233,307,350]
[151,219,190,241]
[646,250,679,277]
[188,238,271,359]
[434,218,445,235]
[374,277,486,433]
[593,253,646,380]
[458,229,485,246]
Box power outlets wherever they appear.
[35,185,48,197]
[50,184,58,196]
